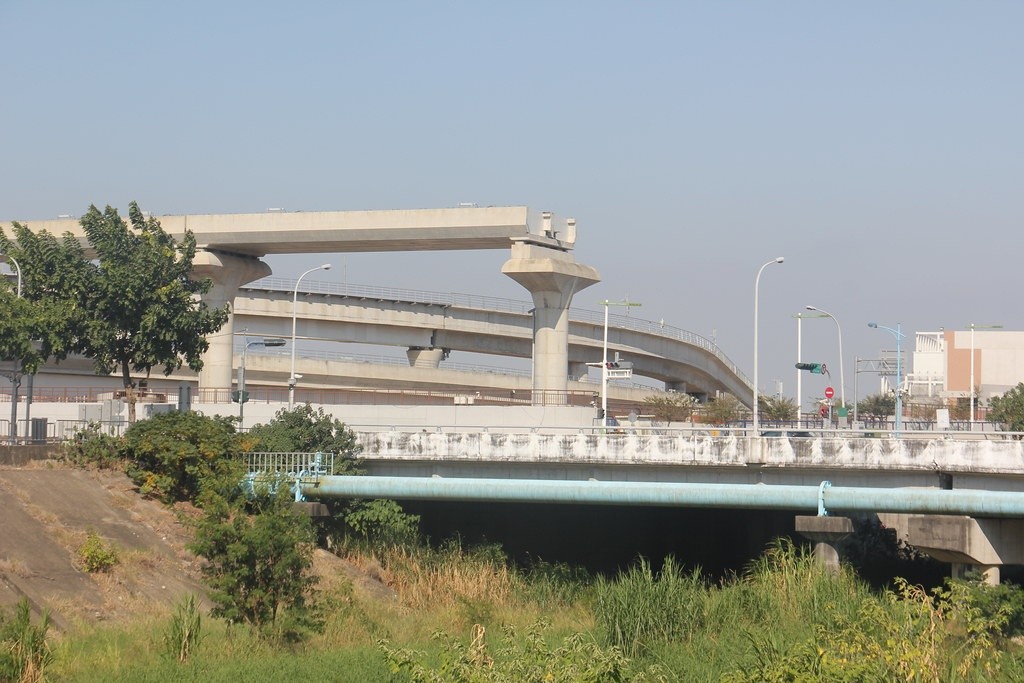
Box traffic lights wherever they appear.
[606,363,619,368]
[796,363,817,370]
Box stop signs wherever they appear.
[825,387,834,398]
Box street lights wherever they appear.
[287,262,331,409]
[806,305,843,408]
[970,323,1003,430]
[602,300,642,426]
[529,308,536,405]
[869,323,900,433]
[753,256,783,432]
[798,312,831,428]
